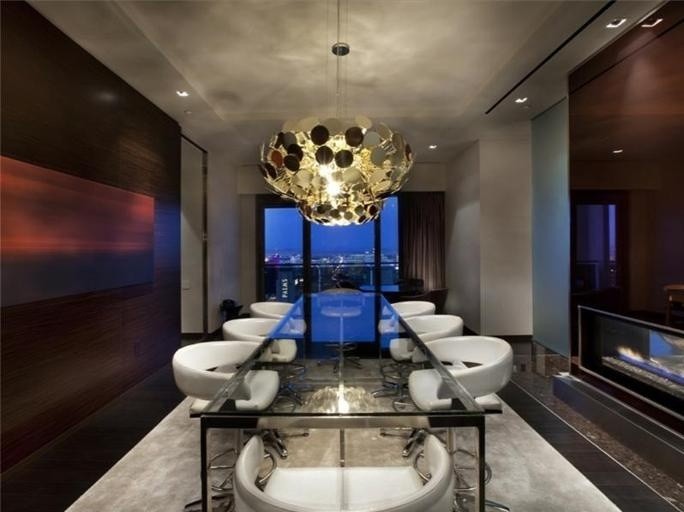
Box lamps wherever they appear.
[256,1,415,228]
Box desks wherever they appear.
[201,292,487,511]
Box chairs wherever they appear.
[373,300,437,396]
[316,289,367,373]
[233,433,455,511]
[172,340,280,509]
[390,313,464,460]
[407,334,516,511]
[224,318,308,458]
[248,302,306,409]
